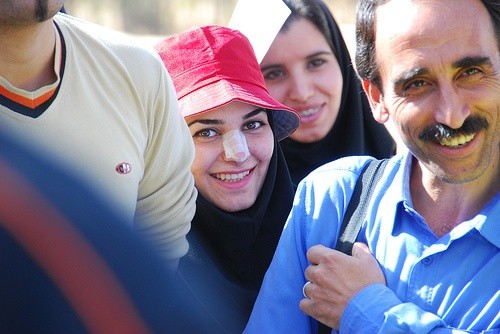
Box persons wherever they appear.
[154,26,301,334]
[259,0,396,188]
[242,0,500,334]
[0,0,198,274]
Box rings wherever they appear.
[303,281,311,299]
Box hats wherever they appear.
[156,25,301,141]
